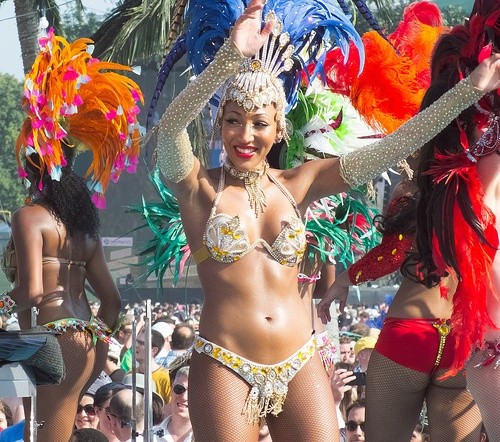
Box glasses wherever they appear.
[173,384,188,394]
[76,404,96,416]
[136,340,145,346]
[99,406,109,415]
[107,413,116,421]
[345,421,365,432]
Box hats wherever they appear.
[85,379,107,395]
[122,374,165,407]
[354,337,377,356]
[151,322,173,338]
[107,337,121,360]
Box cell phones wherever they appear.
[344,372,366,386]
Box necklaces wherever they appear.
[223,159,270,218]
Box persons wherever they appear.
[0,313,21,331]
[69,428,108,442]
[86,300,203,395]
[0,400,13,432]
[317,82,482,442]
[156,324,196,369]
[344,398,365,442]
[94,383,127,442]
[0,132,122,442]
[411,420,429,442]
[0,419,24,442]
[157,0,500,442]
[153,366,194,442]
[430,23,500,442]
[331,303,389,442]
[127,329,171,405]
[75,393,99,429]
[109,389,167,442]
